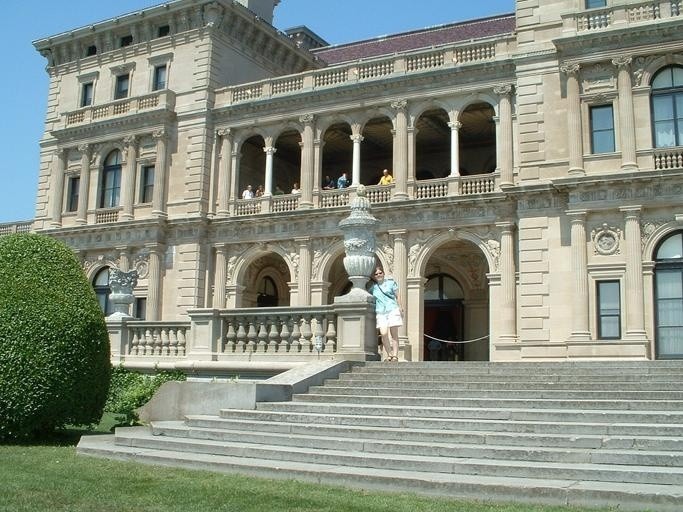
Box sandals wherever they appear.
[384,356,393,363]
[391,355,399,363]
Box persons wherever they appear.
[427,338,442,360]
[272,184,284,211]
[290,182,299,208]
[254,184,264,214]
[337,171,350,189]
[445,341,456,361]
[365,266,404,361]
[322,175,335,189]
[376,168,392,186]
[241,184,254,213]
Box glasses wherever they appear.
[374,272,383,276]
